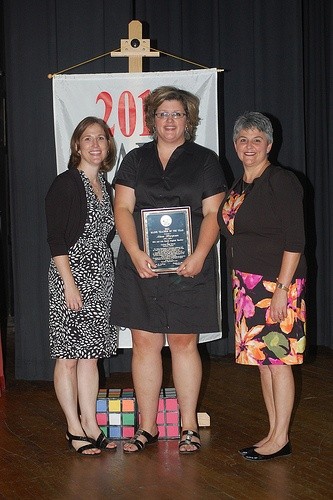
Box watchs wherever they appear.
[276,282,289,292]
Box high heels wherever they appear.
[88,430,117,453]
[65,430,102,457]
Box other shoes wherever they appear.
[239,446,260,456]
[243,440,292,461]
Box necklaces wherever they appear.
[241,159,266,194]
[90,184,99,192]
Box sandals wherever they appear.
[179,430,201,454]
[123,428,159,454]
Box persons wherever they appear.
[47,113,118,455]
[215,111,310,461]
[110,87,228,455]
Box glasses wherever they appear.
[153,112,187,119]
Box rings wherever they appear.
[278,312,283,315]
[192,276,194,278]
